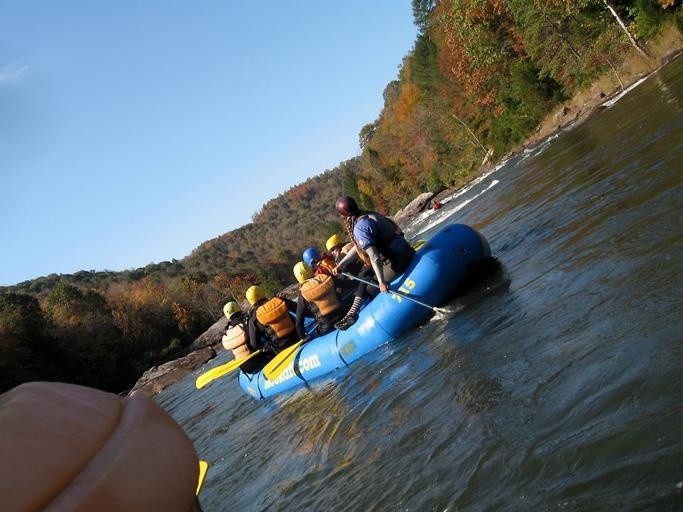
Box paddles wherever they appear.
[196,349,262,390]
[337,270,456,318]
[263,323,319,381]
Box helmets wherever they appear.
[336,198,358,217]
[223,302,240,319]
[246,286,265,305]
[293,261,313,282]
[303,247,321,266]
[326,234,348,251]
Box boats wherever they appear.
[234,220,491,403]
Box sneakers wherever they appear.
[334,314,357,330]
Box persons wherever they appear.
[289,259,359,343]
[241,282,314,354]
[302,248,354,300]
[324,234,363,273]
[333,195,417,295]
[220,300,272,373]
[429,199,443,211]
[330,242,373,332]
